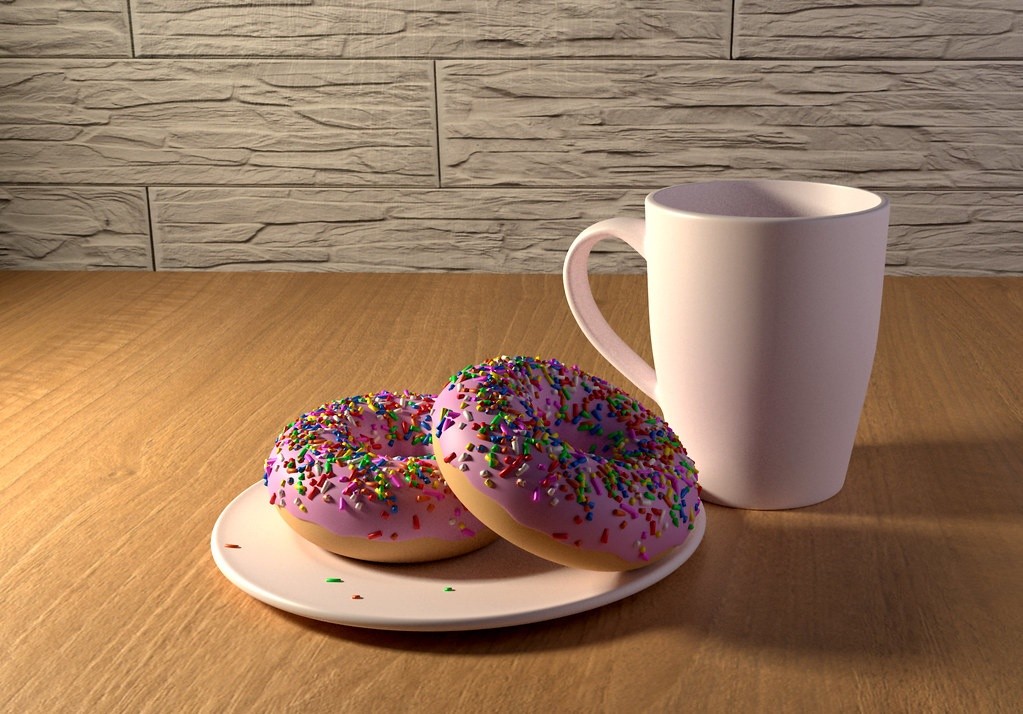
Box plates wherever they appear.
[208,478,706,633]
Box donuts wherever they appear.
[264,387,503,564]
[430,355,702,573]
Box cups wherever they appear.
[561,179,890,512]
